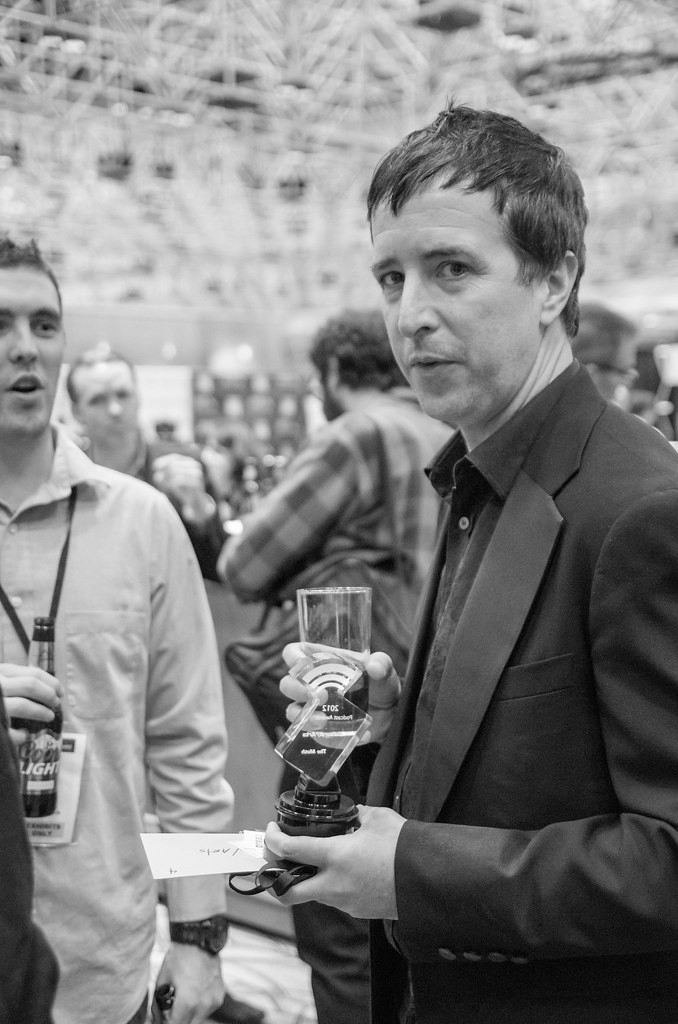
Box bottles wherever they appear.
[153,983,175,1024]
[10,616,64,819]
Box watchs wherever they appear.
[170,916,229,954]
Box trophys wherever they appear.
[258,586,375,900]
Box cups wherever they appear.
[297,585,373,748]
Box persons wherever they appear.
[265,96,677,1024]
[218,309,455,1024]
[0,231,237,1024]
[0,686,62,1024]
[568,302,642,407]
[69,348,297,585]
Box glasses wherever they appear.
[307,367,325,400]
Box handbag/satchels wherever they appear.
[224,561,413,756]
